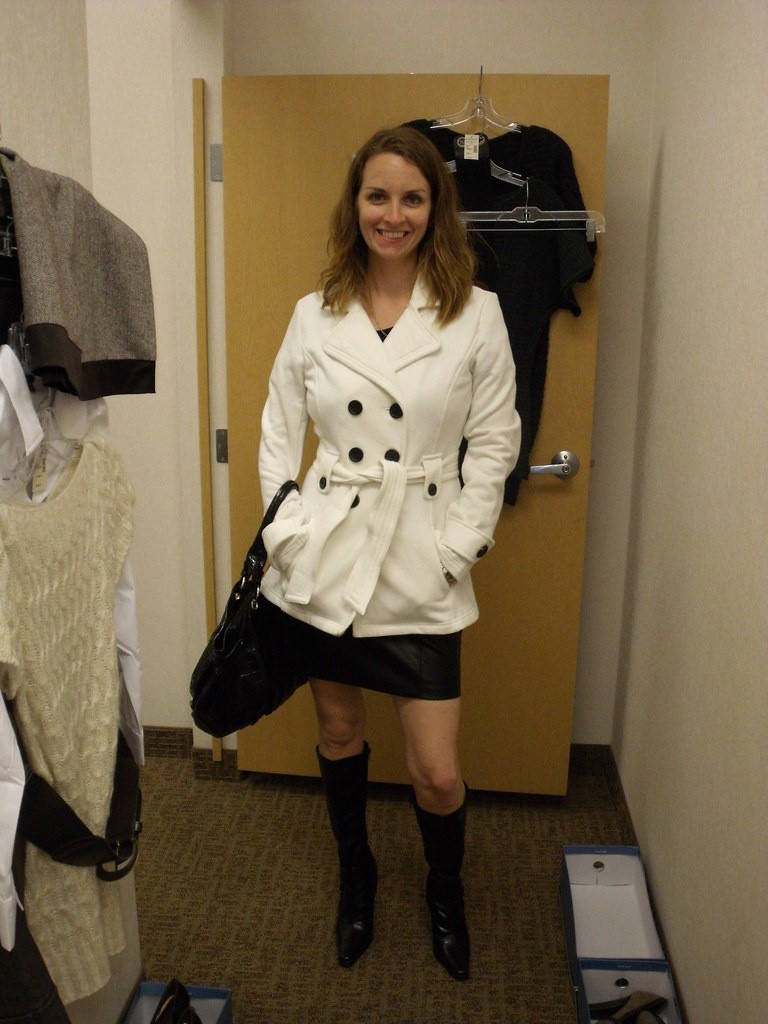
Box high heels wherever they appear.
[589,990,668,1024]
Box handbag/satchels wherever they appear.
[191,479,308,739]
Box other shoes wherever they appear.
[149,978,202,1024]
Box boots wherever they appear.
[410,780,471,981]
[316,741,378,969]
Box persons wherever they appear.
[259,125,521,980]
